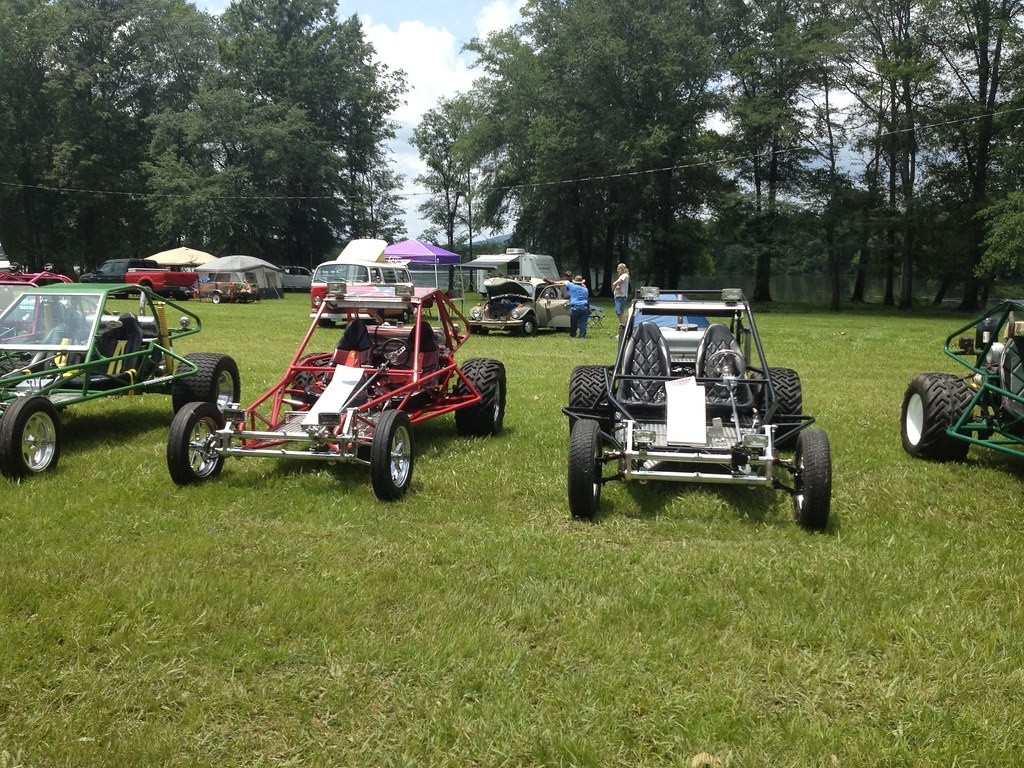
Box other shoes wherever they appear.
[615,334,619,338]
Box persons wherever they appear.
[611,264,631,339]
[543,271,589,339]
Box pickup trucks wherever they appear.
[79,257,198,302]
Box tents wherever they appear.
[140,246,220,266]
[384,239,464,318]
[196,256,283,301]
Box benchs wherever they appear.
[535,288,543,302]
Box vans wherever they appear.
[310,260,415,328]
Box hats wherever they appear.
[572,275,586,284]
[565,271,572,275]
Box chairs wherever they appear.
[385,272,396,283]
[22,310,144,389]
[588,305,605,329]
[616,322,671,409]
[998,336,1024,418]
[305,318,374,407]
[696,324,756,412]
[445,289,463,320]
[416,298,433,319]
[375,321,439,402]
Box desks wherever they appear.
[422,298,465,322]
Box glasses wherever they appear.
[565,275,570,277]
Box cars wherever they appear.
[468,276,571,338]
[278,265,314,293]
